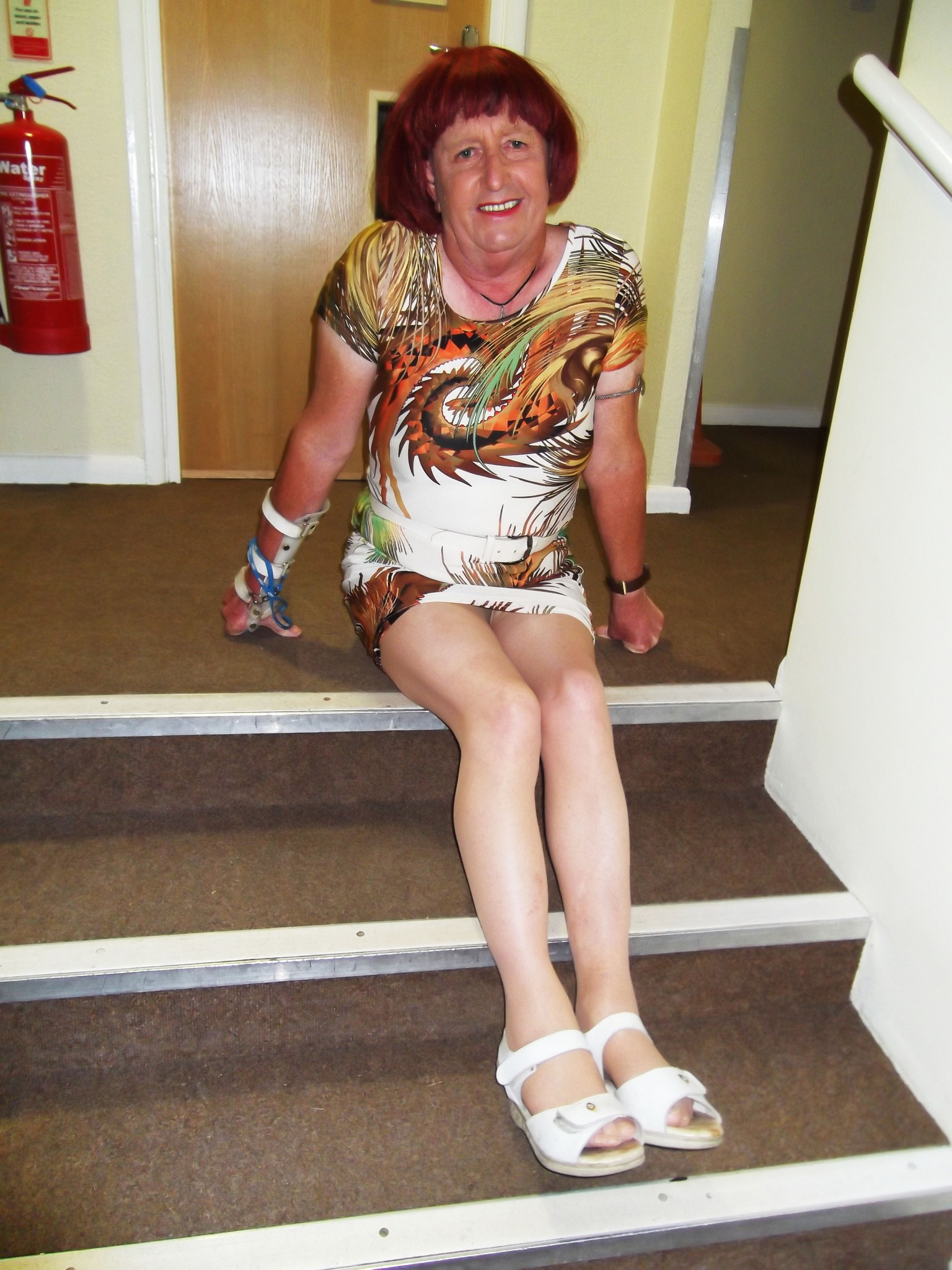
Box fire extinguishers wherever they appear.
[1,64,93,358]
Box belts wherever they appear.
[370,492,559,565]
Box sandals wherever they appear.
[492,1026,648,1176]
[582,1011,724,1150]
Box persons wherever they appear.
[220,45,722,1176]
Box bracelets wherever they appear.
[605,562,650,596]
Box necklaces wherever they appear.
[480,266,537,319]
